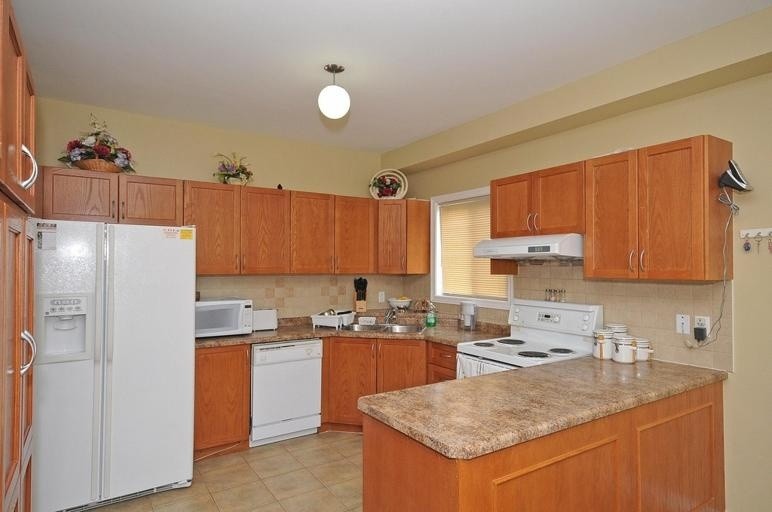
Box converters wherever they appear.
[694,327,706,340]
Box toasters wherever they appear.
[253,309,279,332]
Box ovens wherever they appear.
[455,353,519,379]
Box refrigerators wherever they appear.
[30,217,196,512]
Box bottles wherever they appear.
[592,324,651,364]
[426,303,437,328]
[544,288,566,303]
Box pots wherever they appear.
[319,308,352,319]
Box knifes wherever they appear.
[353,277,367,301]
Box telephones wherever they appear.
[719,160,752,191]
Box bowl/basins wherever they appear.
[387,297,412,310]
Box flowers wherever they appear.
[368,172,403,199]
[58,118,139,170]
[213,152,254,186]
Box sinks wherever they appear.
[340,323,388,332]
[382,325,428,334]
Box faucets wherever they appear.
[383,309,396,324]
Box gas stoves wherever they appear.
[457,326,592,367]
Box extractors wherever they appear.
[473,233,584,261]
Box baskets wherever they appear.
[225,176,247,186]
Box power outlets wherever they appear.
[693,315,712,338]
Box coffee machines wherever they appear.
[456,301,478,333]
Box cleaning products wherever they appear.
[426,300,438,327]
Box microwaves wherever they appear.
[195,296,253,339]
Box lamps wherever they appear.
[318,63,352,122]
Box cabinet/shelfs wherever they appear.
[40,168,185,223]
[0,191,34,511]
[185,183,290,275]
[1,1,37,218]
[194,344,252,462]
[490,160,586,238]
[323,336,429,431]
[428,340,456,385]
[291,191,373,277]
[583,135,736,283]
[375,199,432,275]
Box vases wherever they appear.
[225,175,249,187]
